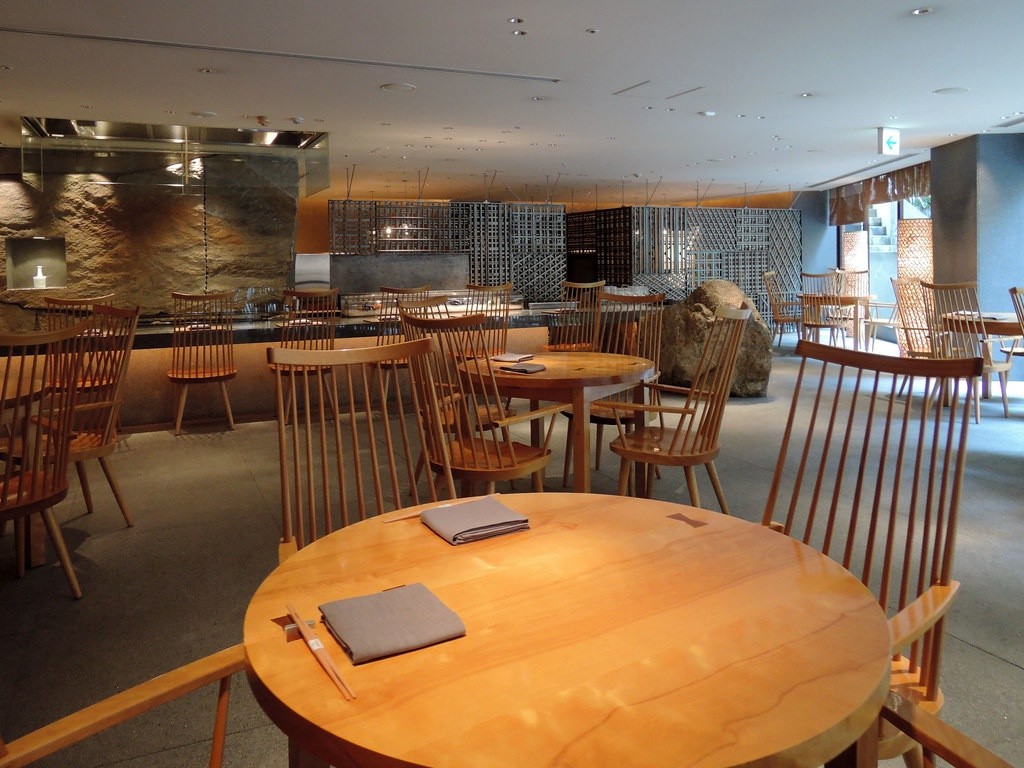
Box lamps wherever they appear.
[380,221,415,240]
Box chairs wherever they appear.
[0,269,1024,768]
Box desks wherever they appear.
[237,496,896,768]
[941,310,1024,407]
[0,375,52,478]
[0,311,549,437]
[796,293,877,354]
[457,351,656,494]
[0,305,670,458]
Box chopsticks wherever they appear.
[285,600,357,702]
[493,369,531,376]
[383,493,503,523]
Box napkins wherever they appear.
[317,584,466,665]
[421,496,529,546]
[490,352,533,362]
[500,363,547,375]
